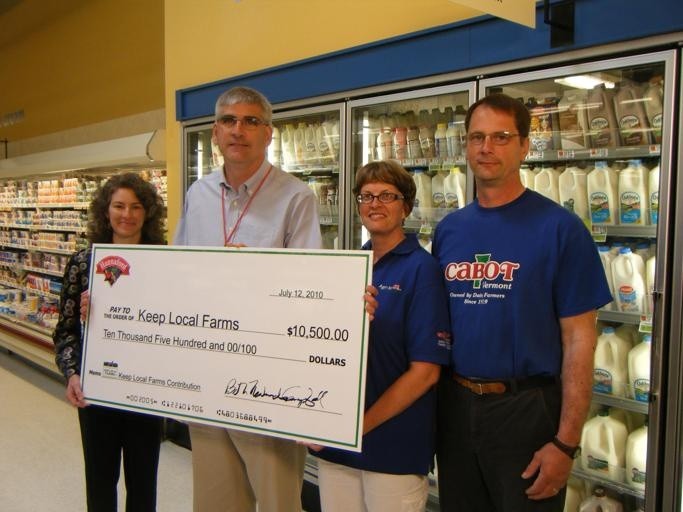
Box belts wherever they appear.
[442,369,557,398]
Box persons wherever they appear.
[429,92,613,512]
[50,171,166,512]
[78,87,378,512]
[298,160,448,512]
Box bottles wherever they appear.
[391,126,401,160]
[376,129,386,161]
[404,127,418,160]
[445,123,460,158]
[436,123,445,156]
[419,124,434,158]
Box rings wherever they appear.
[552,486,558,494]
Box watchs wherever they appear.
[550,434,582,460]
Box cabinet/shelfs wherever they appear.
[0,157,168,389]
[478,48,678,512]
[346,80,480,512]
[178,99,347,492]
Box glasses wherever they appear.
[355,192,406,205]
[466,130,523,146]
[214,116,269,129]
